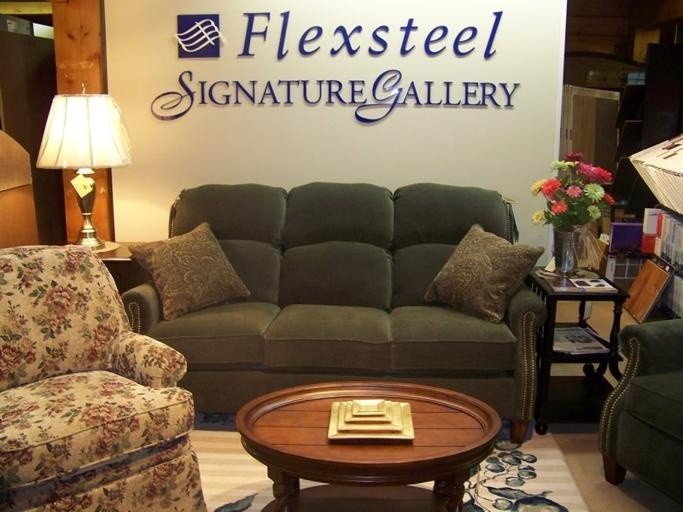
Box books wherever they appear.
[566,345,610,356]
[552,326,604,352]
[545,276,618,294]
[608,206,682,324]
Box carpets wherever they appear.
[188,410,589,512]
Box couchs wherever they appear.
[122,183,545,447]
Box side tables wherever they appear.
[526,266,630,434]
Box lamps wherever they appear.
[37,84,133,252]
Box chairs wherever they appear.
[0,243,214,512]
[600,319,683,505]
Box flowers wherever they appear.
[529,153,616,232]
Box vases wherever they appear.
[559,233,577,274]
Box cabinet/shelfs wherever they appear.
[61,244,142,294]
[560,83,622,173]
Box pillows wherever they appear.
[422,221,544,326]
[127,219,251,320]
